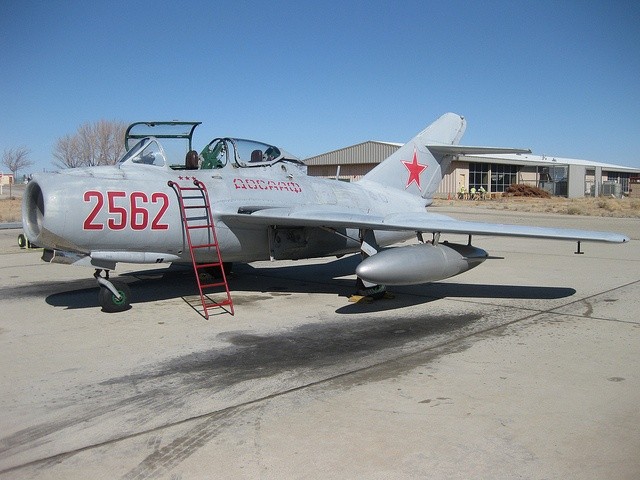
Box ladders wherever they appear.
[168,179,234,320]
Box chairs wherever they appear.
[186,150,198,169]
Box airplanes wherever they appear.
[18,112,630,320]
